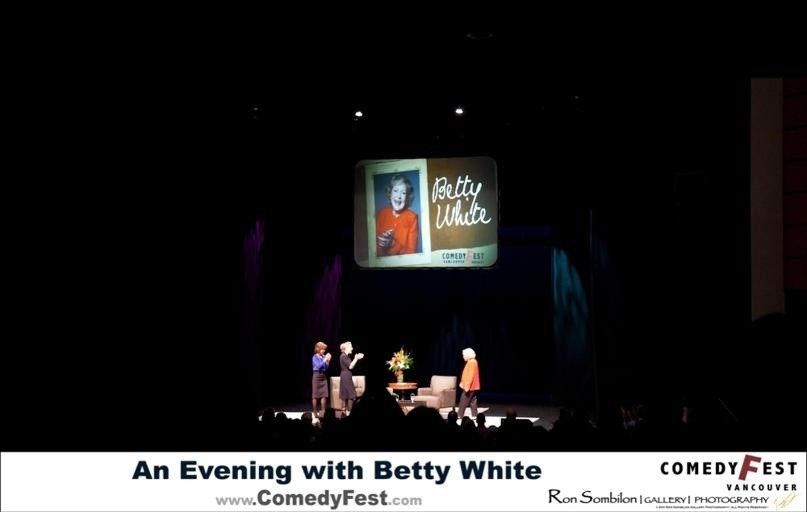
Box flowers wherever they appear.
[385,344,415,376]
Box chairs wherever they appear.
[329,375,367,410]
[417,375,457,408]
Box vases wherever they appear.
[397,374,403,383]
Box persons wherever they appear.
[339,342,365,416]
[237,388,715,451]
[455,345,481,422]
[374,174,418,257]
[310,340,332,421]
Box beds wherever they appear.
[387,382,417,400]
